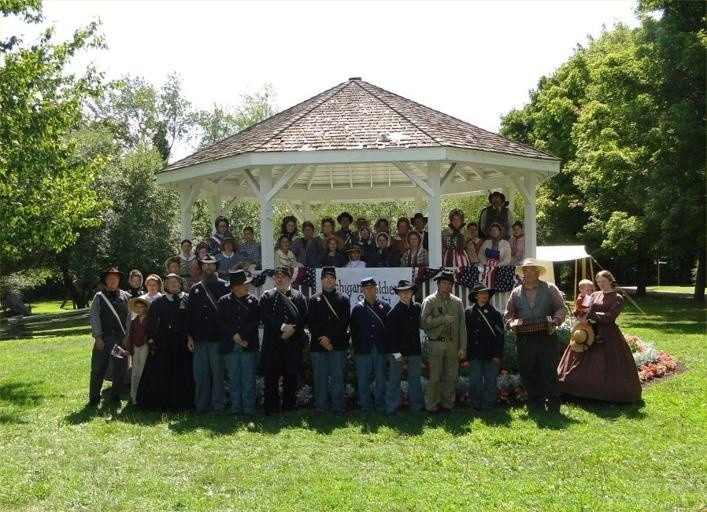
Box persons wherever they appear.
[216,269,259,415]
[384,280,424,418]
[419,270,469,413]
[274,212,428,268]
[87,257,163,407]
[557,269,641,403]
[135,273,194,414]
[502,258,568,411]
[258,264,308,415]
[164,215,261,286]
[307,268,350,414]
[183,251,231,416]
[465,282,505,411]
[442,191,524,267]
[574,279,605,344]
[349,277,391,413]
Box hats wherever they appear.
[468,283,497,303]
[222,230,235,243]
[321,212,428,254]
[127,294,151,315]
[570,322,594,353]
[514,258,546,277]
[448,192,510,237]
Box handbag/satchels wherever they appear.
[484,248,499,259]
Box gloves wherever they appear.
[393,353,402,363]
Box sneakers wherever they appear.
[595,336,604,344]
